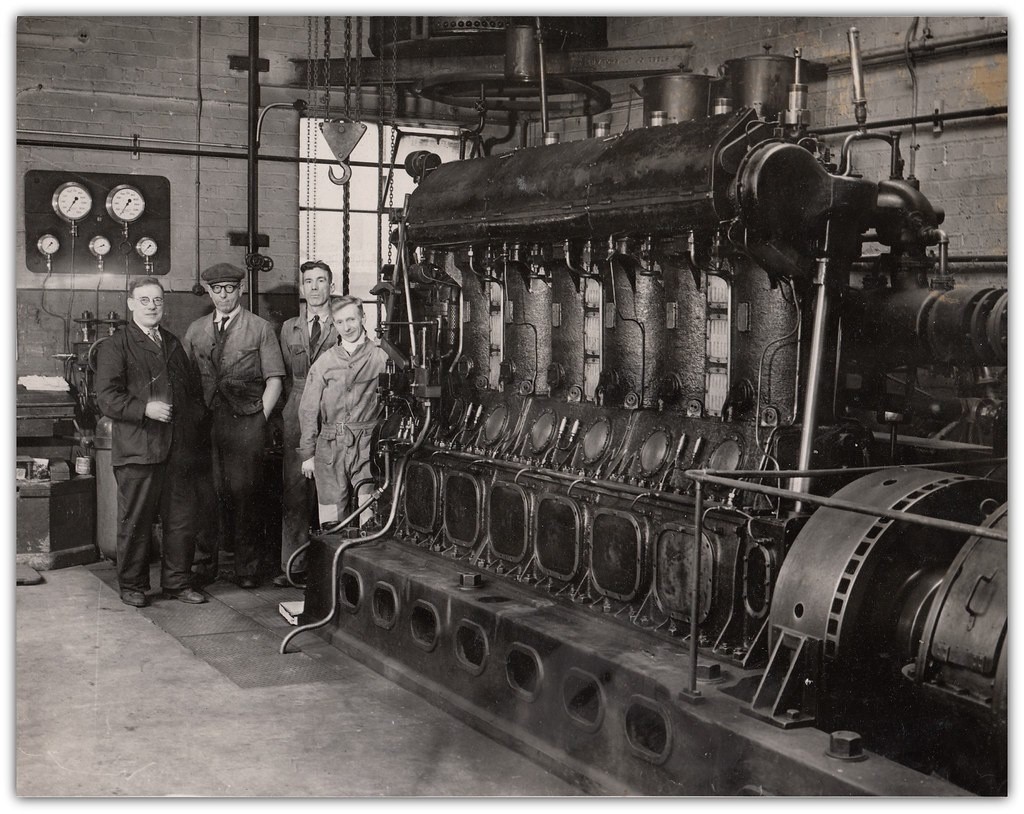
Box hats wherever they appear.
[201,263,245,284]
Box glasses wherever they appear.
[130,297,164,306]
[210,281,241,294]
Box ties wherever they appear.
[149,329,162,347]
[310,315,321,357]
[219,317,229,337]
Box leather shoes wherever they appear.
[121,590,146,606]
[163,588,205,603]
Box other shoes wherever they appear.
[188,574,216,589]
[239,575,256,588]
[274,573,305,587]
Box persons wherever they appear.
[96,276,206,606]
[295,295,394,534]
[272,260,342,587]
[184,263,286,588]
